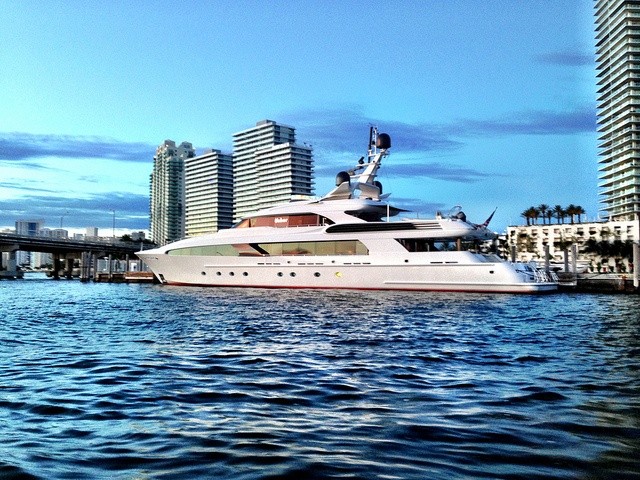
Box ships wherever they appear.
[134,123,560,292]
